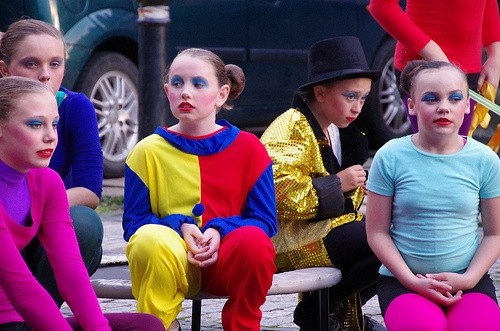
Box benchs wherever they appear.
[87,262,342,331]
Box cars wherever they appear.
[1,0,499,180]
